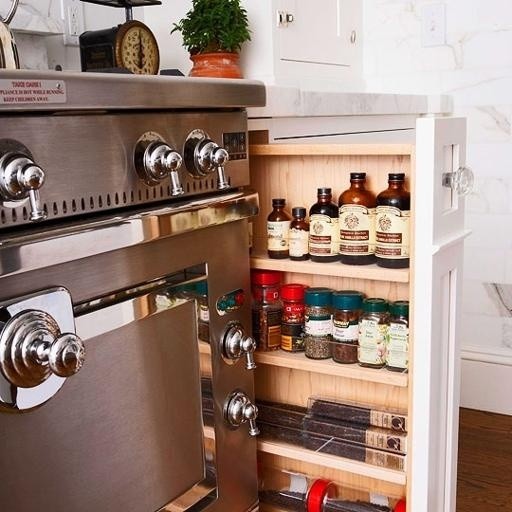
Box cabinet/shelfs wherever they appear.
[124,114,475,511]
[274,1,365,68]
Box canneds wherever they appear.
[252,271,409,372]
[148,280,210,344]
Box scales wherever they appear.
[79,0,186,76]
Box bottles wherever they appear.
[251,172,410,267]
[251,268,409,374]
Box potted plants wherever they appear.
[170,1,252,80]
